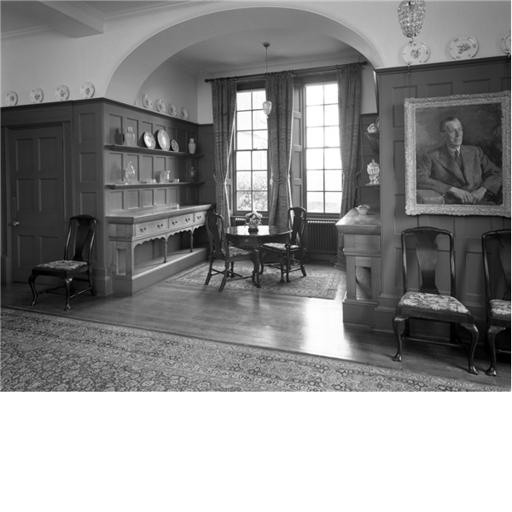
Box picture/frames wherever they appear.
[399,89,510,221]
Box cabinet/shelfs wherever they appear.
[353,112,380,211]
[101,141,206,191]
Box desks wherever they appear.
[333,204,381,303]
[106,200,214,278]
[221,223,295,287]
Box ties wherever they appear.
[453,148,464,172]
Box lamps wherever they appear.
[256,39,280,117]
[396,1,430,48]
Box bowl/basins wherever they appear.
[355,205,369,216]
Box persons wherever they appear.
[416,115,502,205]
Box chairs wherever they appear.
[26,213,100,312]
[472,226,510,378]
[204,210,260,294]
[388,224,482,378]
[261,204,308,284]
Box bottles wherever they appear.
[366,160,379,184]
[188,138,197,155]
[125,127,137,146]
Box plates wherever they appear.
[179,106,188,119]
[450,32,479,59]
[144,131,156,149]
[79,81,95,98]
[500,30,511,54]
[55,85,69,100]
[142,94,153,108]
[32,88,43,103]
[6,90,17,107]
[157,128,170,151]
[156,99,166,112]
[401,42,429,65]
[166,103,176,115]
[171,139,180,152]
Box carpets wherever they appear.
[0,298,510,392]
[164,255,342,303]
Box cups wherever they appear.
[160,170,171,183]
